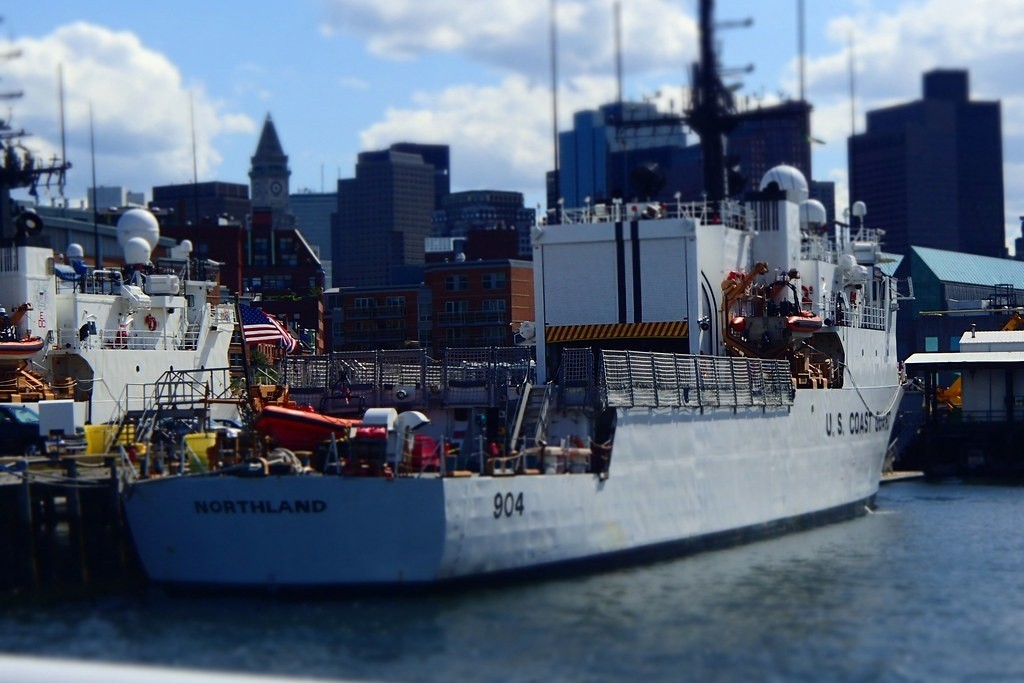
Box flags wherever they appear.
[238,302,302,357]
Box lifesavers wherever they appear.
[801,284,810,305]
[396,390,407,400]
[148,317,157,331]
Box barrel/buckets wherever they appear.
[184,433,216,472]
[85,425,115,453]
[113,424,134,445]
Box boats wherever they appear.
[0,48,272,484]
[903,308,1024,482]
[123,0,907,599]
[255,403,364,451]
